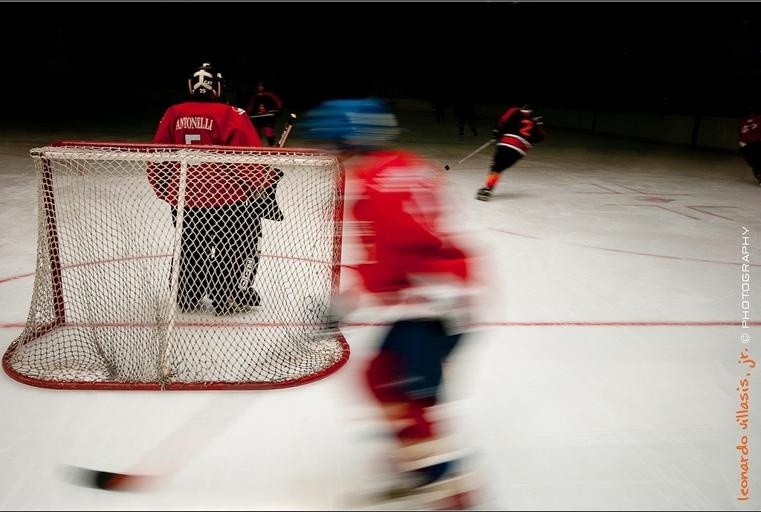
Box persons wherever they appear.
[737,110,760,185]
[148,58,275,312]
[245,80,282,144]
[301,99,487,509]
[474,102,544,202]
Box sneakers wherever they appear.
[478,188,491,196]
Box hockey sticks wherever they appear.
[52,296,349,493]
[444,139,498,171]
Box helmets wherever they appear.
[182,61,227,100]
